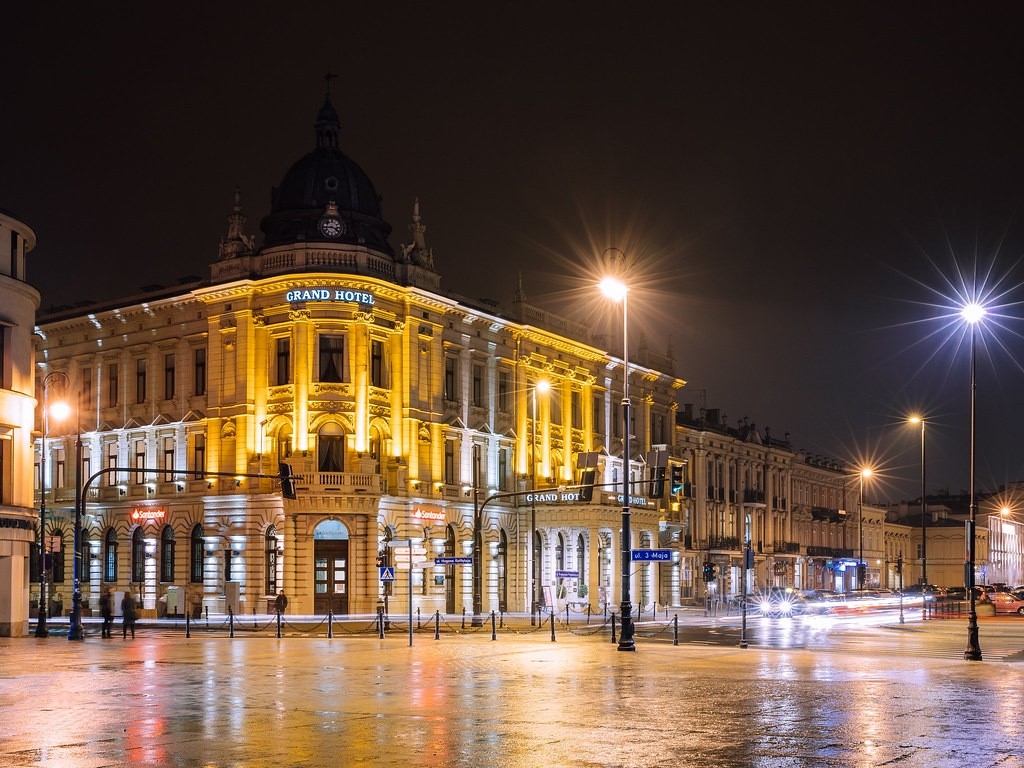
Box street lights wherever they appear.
[530,377,551,626]
[33,370,71,638]
[958,304,985,661]
[858,467,873,590]
[907,414,927,584]
[597,248,638,651]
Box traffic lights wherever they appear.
[708,562,716,581]
[702,561,710,583]
[670,463,684,496]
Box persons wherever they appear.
[273,589,291,630]
[95,584,115,640]
[118,588,139,640]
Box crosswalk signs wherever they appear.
[379,567,395,582]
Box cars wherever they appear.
[732,582,1024,617]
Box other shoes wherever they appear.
[101,635,115,639]
[123,637,126,639]
[276,623,284,628]
